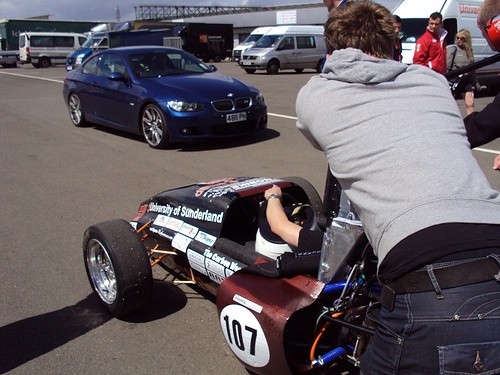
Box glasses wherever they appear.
[457,36,465,40]
[430,23,438,25]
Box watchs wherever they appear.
[267,194,281,202]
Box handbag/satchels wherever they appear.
[448,76,460,92]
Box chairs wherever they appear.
[152,54,168,72]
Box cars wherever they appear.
[63,45,269,150]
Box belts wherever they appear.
[381,256,500,316]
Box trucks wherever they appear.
[64,21,174,72]
[390,0,500,76]
[0,14,108,68]
[170,22,234,63]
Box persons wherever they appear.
[446,28,480,116]
[263,183,326,278]
[295,0,500,375]
[413,11,448,75]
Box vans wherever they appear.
[232,25,282,63]
[238,25,328,75]
[19,31,88,69]
